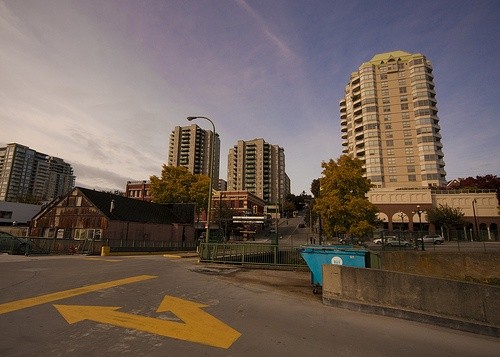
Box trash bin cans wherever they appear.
[299,247,370,295]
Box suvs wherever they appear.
[417,234,444,246]
[372,236,396,245]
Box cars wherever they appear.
[386,237,411,247]
[339,234,365,245]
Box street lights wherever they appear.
[376,216,385,251]
[299,202,312,238]
[276,201,279,245]
[186,116,215,263]
[411,205,426,251]
[398,212,407,241]
